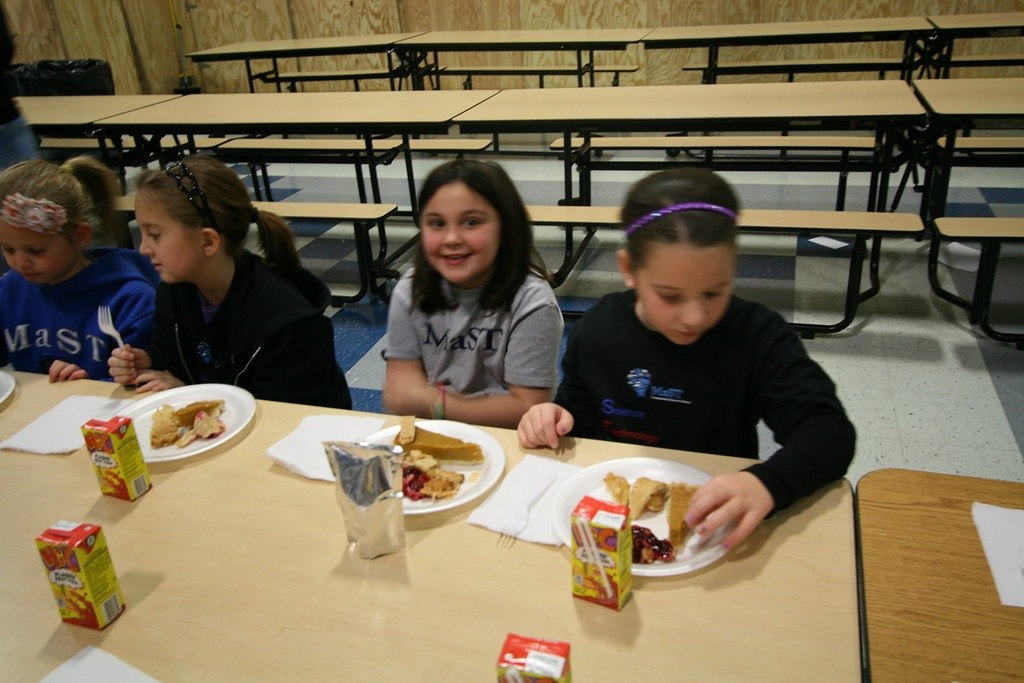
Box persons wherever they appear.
[0,0,38,171]
[518,168,856,550]
[108,158,354,411]
[381,160,563,429]
[1,156,162,383]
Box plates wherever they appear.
[115,383,256,463]
[0,370,15,403]
[357,419,505,514]
[550,457,731,576]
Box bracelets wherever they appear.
[436,384,445,420]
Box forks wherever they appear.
[98,306,138,387]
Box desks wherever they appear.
[453,82,927,288]
[40,88,502,293]
[185,12,1023,80]
[11,94,180,166]
[1,371,863,682]
[852,468,1023,683]
[911,77,1022,324]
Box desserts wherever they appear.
[388,414,484,499]
[149,399,224,450]
[603,471,700,564]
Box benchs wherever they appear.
[37,57,1023,353]
